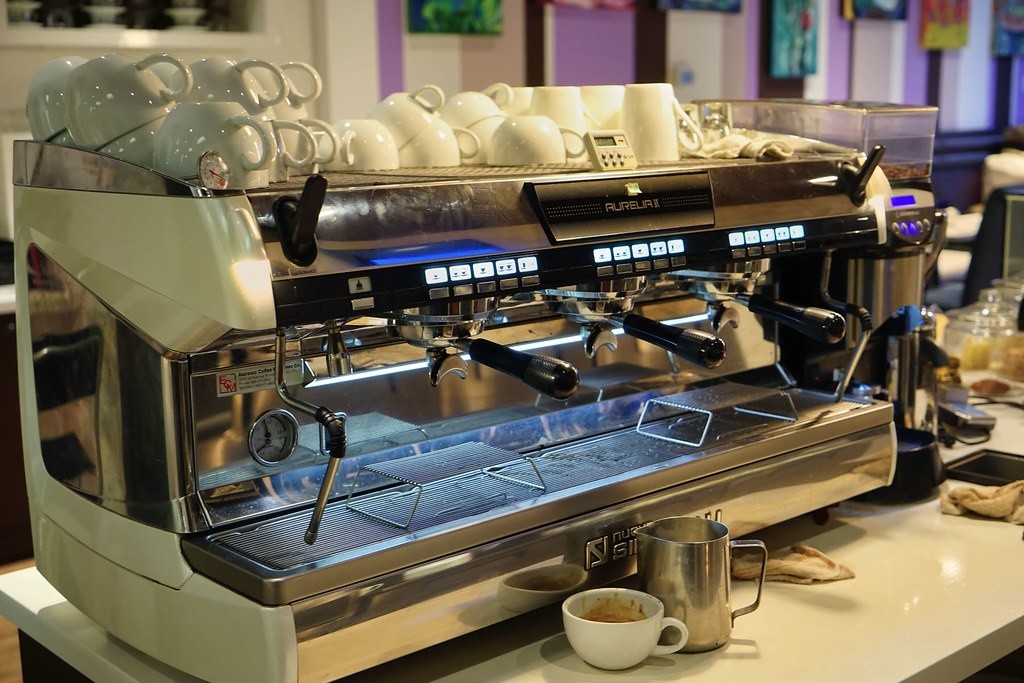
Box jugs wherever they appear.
[566,512,646,586]
[633,516,768,654]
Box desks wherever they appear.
[0,385,1024,683]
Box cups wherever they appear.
[64,52,194,168]
[251,61,322,121]
[562,588,688,670]
[258,120,318,182]
[173,55,289,121]
[495,87,534,115]
[26,56,90,147]
[440,83,514,136]
[494,562,587,619]
[398,119,481,168]
[152,101,277,189]
[318,118,400,170]
[623,83,705,162]
[534,86,602,162]
[487,114,587,166]
[280,118,341,177]
[458,116,507,164]
[367,85,446,150]
[581,85,625,131]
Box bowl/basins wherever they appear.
[945,289,1024,396]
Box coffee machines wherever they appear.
[695,97,948,506]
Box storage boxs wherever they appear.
[691,96,941,181]
